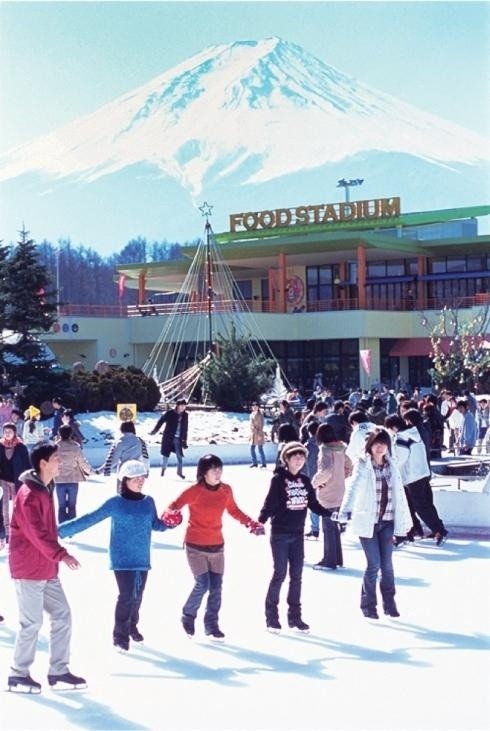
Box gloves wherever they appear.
[336,523,347,533]
[161,510,182,528]
[250,521,264,535]
[331,511,348,523]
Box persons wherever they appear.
[60,411,83,437]
[0,426,31,545]
[4,410,21,426]
[401,409,441,540]
[50,428,90,524]
[21,405,45,445]
[255,441,341,634]
[54,460,175,655]
[6,441,88,695]
[381,412,449,548]
[301,419,323,541]
[161,455,264,641]
[335,428,412,621]
[269,384,490,453]
[50,399,69,438]
[94,421,151,493]
[310,423,354,572]
[148,398,187,481]
[247,404,267,468]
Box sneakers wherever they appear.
[183,620,194,635]
[437,532,448,545]
[305,531,319,537]
[48,673,86,685]
[9,676,41,688]
[205,629,224,637]
[336,562,342,566]
[288,619,309,629]
[313,561,336,570]
[384,605,400,617]
[267,617,281,629]
[130,626,143,641]
[365,608,379,619]
[114,635,129,650]
[408,527,423,536]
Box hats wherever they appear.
[280,442,309,464]
[24,409,30,420]
[30,405,41,418]
[176,398,188,405]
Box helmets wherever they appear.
[118,460,147,482]
